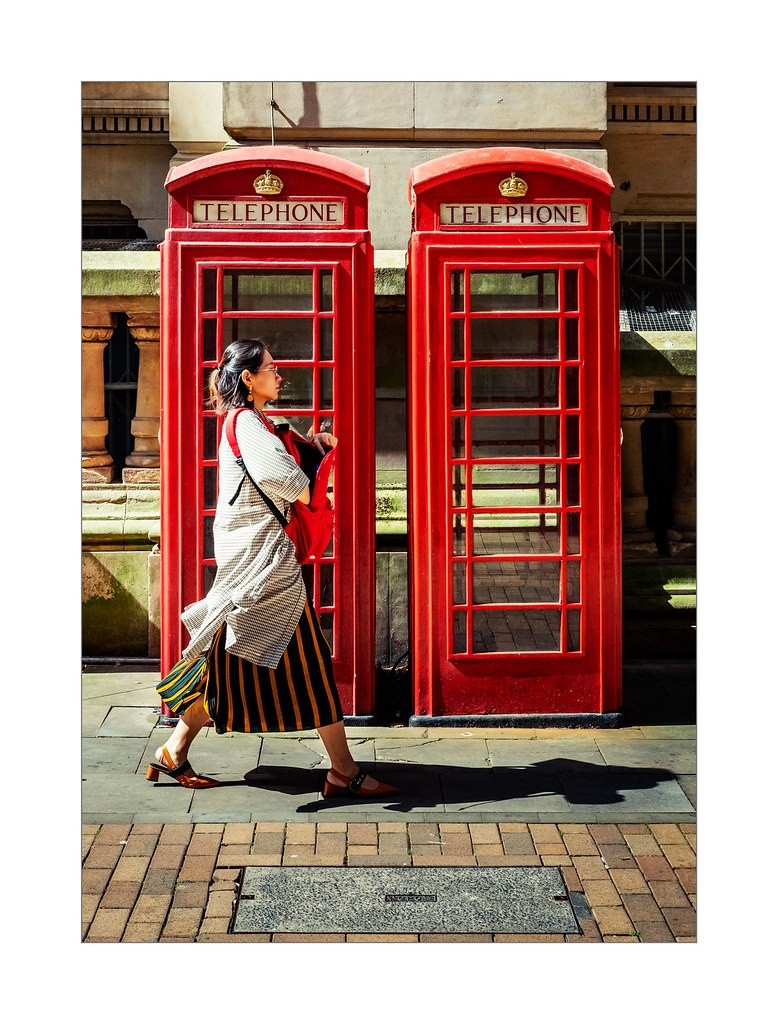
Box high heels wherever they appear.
[146,745,220,788]
[324,767,399,798]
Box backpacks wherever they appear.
[227,407,336,565]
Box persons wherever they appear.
[146,339,399,799]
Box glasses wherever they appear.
[257,365,277,375]
